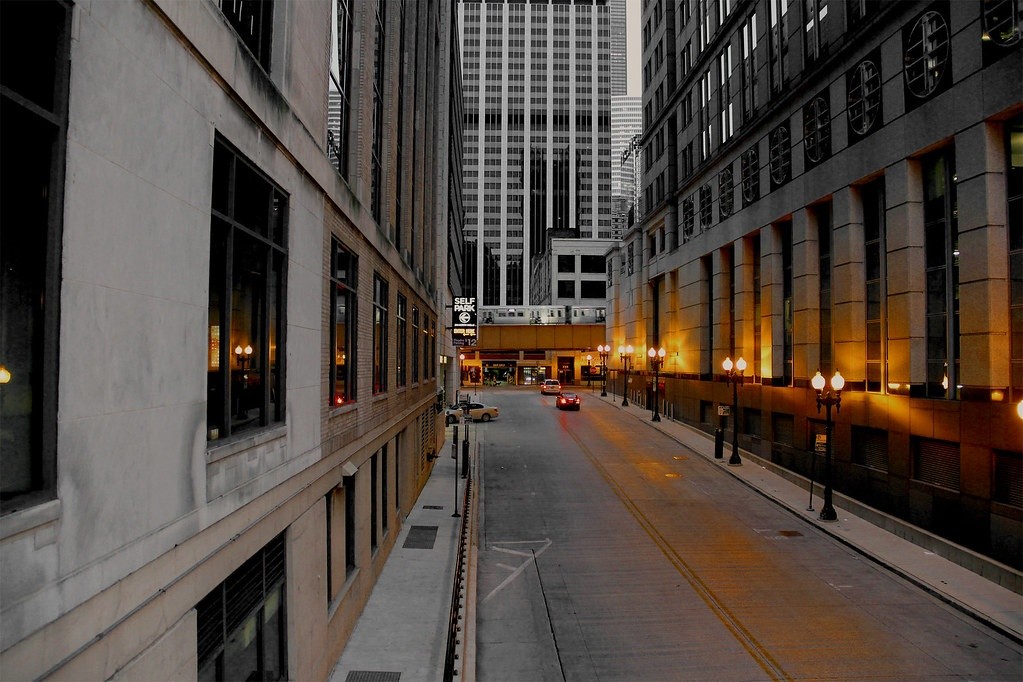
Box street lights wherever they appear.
[597,345,610,396]
[586,354,593,386]
[812,368,845,521]
[617,344,633,407]
[648,347,666,422]
[460,354,465,387]
[722,354,747,466]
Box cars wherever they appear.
[555,392,581,410]
[540,379,561,394]
[444,401,499,424]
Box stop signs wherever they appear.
[590,367,597,374]
